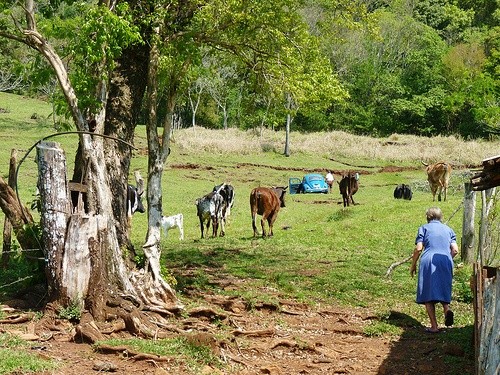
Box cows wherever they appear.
[212,183,235,226]
[250,186,288,240]
[196,192,225,239]
[129,184,146,215]
[337,176,358,207]
[421,160,453,202]
[161,214,183,240]
[394,184,413,200]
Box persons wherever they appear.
[326,170,334,193]
[410,208,460,331]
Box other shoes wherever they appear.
[445,310,454,326]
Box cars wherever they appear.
[289,173,329,195]
